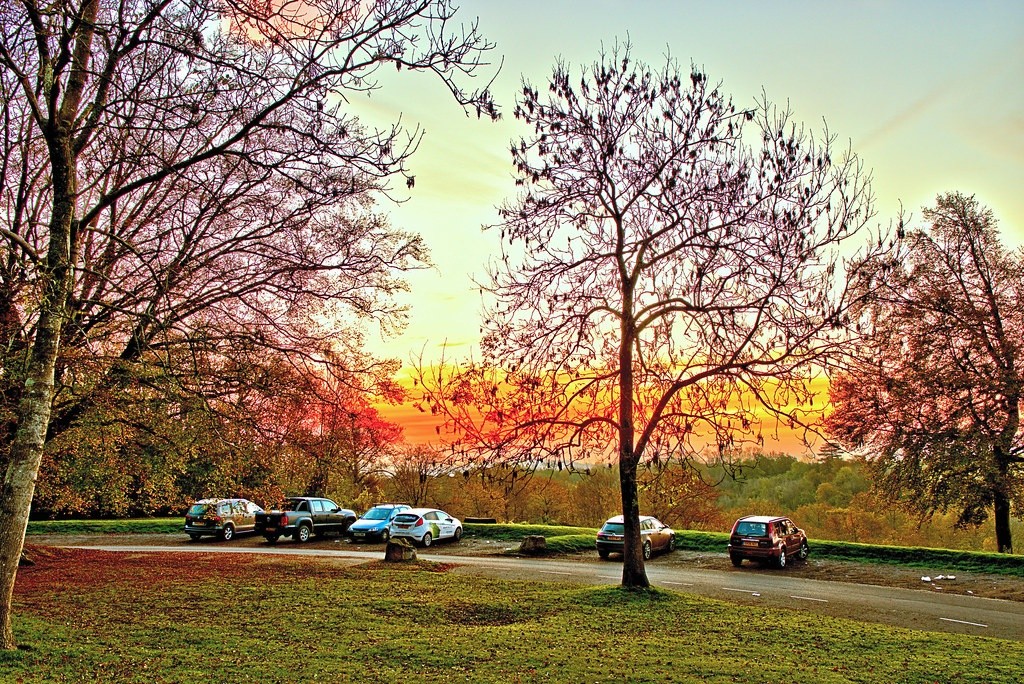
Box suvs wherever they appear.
[389,507,463,548]
[597,514,676,562]
[184,499,264,542]
[728,515,810,570]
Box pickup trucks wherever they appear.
[254,496,358,545]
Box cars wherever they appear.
[346,501,412,544]
